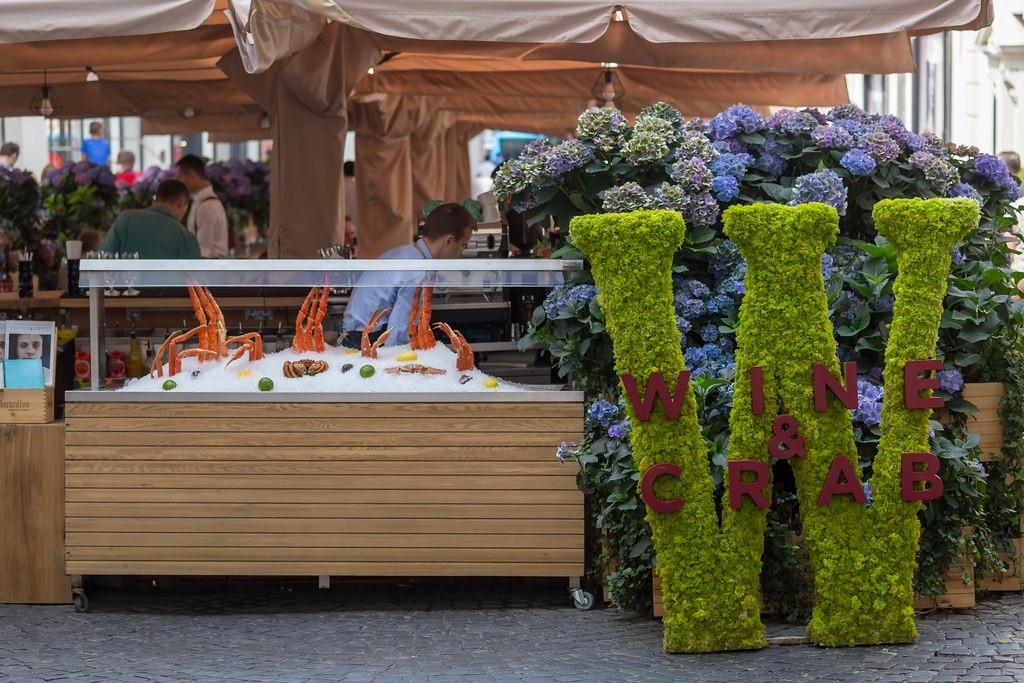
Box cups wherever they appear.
[85,252,102,297]
[121,251,141,296]
[101,252,121,298]
[509,323,524,339]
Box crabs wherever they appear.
[150,270,475,384]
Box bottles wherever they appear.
[127,332,143,379]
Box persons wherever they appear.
[78,229,103,256]
[0,334,5,361]
[80,122,111,166]
[997,151,1021,177]
[100,179,201,259]
[340,203,479,350]
[344,161,357,245]
[114,151,137,186]
[17,335,49,384]
[1002,196,1024,273]
[176,154,229,259]
[0,143,19,172]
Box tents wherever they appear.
[0,0,996,258]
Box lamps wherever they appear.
[28,68,64,120]
[590,67,626,102]
